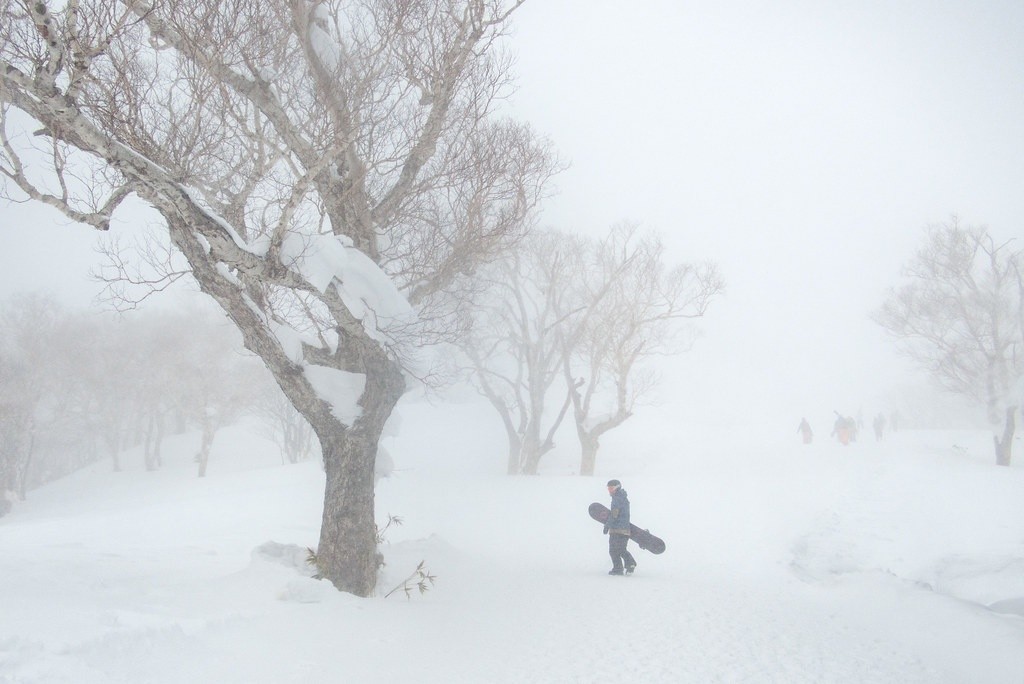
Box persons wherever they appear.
[602,480,637,576]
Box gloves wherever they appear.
[603,526,609,534]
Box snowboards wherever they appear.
[587,501,667,555]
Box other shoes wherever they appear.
[627,565,635,575]
[609,569,623,575]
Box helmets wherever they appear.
[607,480,622,490]
